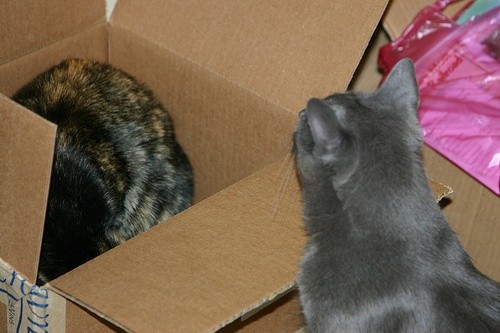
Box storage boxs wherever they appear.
[1,0,500,331]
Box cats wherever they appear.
[10,57,195,288]
[292,58,500,333]
